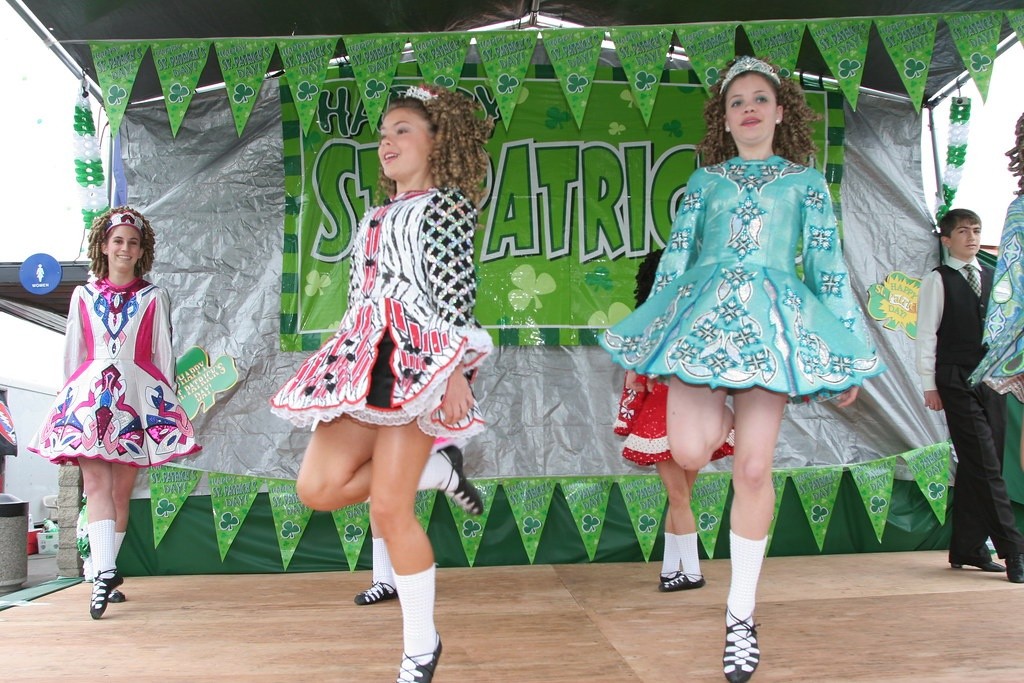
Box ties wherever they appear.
[962,264,981,297]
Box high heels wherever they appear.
[1004,553,1024,583]
[951,555,1006,572]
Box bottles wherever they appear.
[28,514,34,532]
[41,518,59,534]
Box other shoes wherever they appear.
[107,589,125,603]
[354,581,398,605]
[397,632,443,683]
[660,570,683,583]
[90,568,124,620]
[723,604,761,683]
[658,574,705,592]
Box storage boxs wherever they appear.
[36,532,59,555]
[28,529,42,555]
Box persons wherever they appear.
[267,87,496,683]
[28,206,202,620]
[599,55,886,683]
[613,247,735,592]
[966,109,1024,403]
[916,208,1024,584]
[354,505,398,605]
